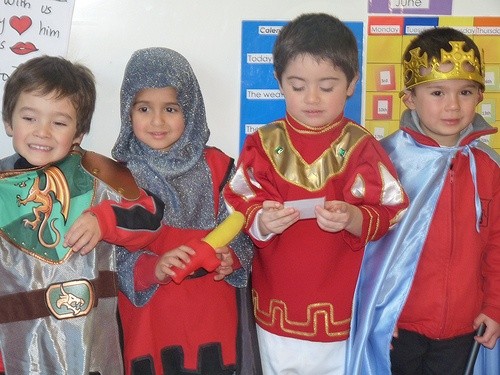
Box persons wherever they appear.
[0,56,166,375]
[344,28,500,375]
[223,13,409,375]
[111,47,254,375]
